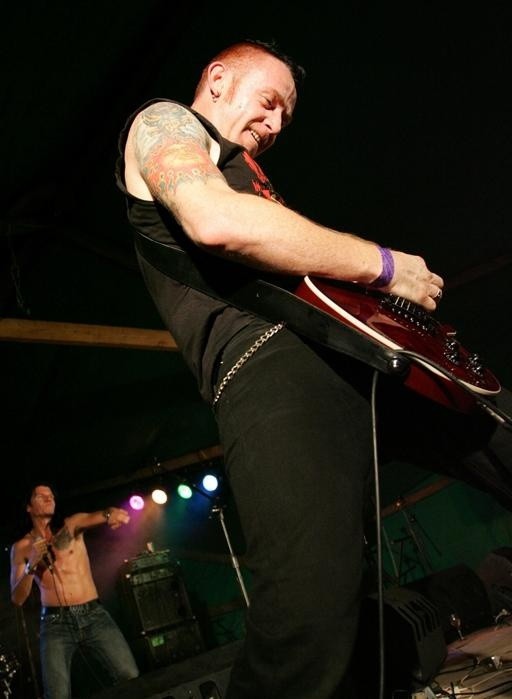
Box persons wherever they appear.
[10,481,142,698]
[115,37,444,698]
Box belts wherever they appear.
[41,599,100,617]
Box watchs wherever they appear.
[104,506,112,525]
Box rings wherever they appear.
[433,287,443,301]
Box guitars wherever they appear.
[291,272,501,413]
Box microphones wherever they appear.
[35,536,53,569]
[152,458,161,472]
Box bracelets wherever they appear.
[370,242,396,287]
[25,562,37,575]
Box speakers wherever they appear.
[94,640,245,699]
[143,621,207,672]
[130,574,196,634]
[329,588,449,699]
[401,563,493,645]
[465,546,512,619]
